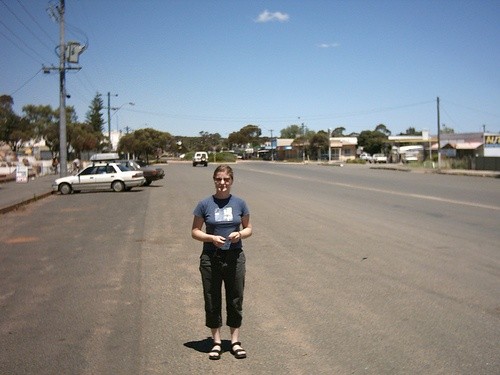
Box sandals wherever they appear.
[230,342,246,359]
[209,341,224,360]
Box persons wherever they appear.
[54,159,59,174]
[192,164,252,359]
[73,162,79,173]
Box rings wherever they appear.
[236,237,238,239]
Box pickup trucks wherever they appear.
[192,151,209,167]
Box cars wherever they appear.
[51,161,147,195]
[402,156,418,165]
[368,153,388,164]
[102,161,165,188]
[1,159,42,182]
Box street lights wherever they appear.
[108,101,135,153]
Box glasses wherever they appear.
[216,178,230,182]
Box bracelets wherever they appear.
[238,232,241,238]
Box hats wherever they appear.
[215,238,238,275]
